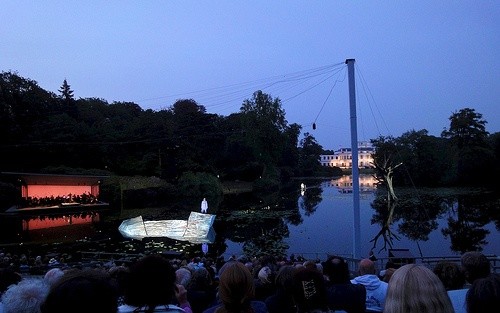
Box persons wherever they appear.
[0,250,500,313]
[201,198,208,214]
[32,192,96,207]
